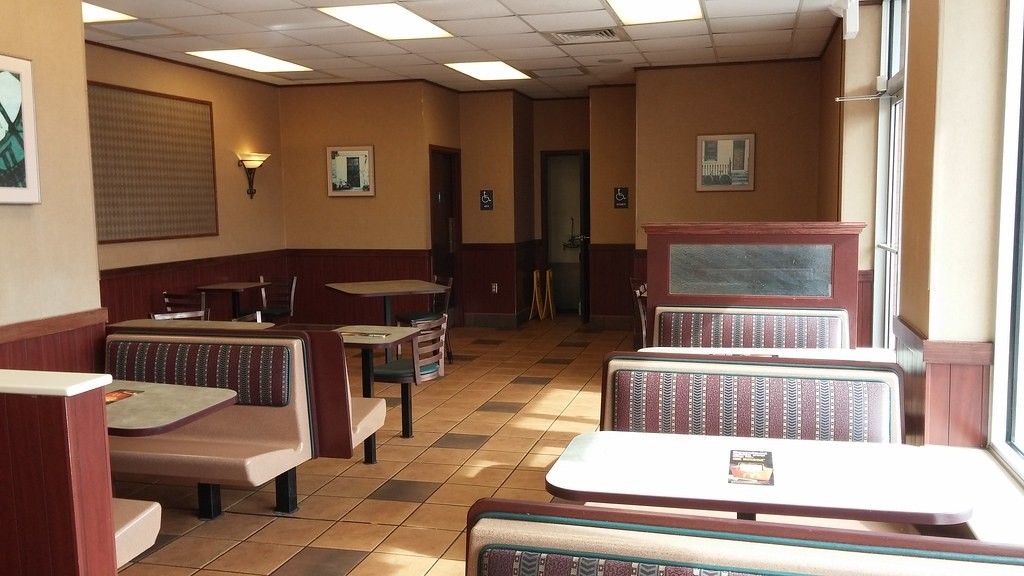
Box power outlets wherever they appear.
[489,283,498,293]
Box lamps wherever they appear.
[235,153,272,199]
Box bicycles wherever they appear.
[703,169,732,185]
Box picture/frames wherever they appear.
[326,145,376,197]
[0,53,41,206]
[695,133,756,192]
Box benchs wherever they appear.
[648,303,850,348]
[0,369,162,571]
[598,350,907,445]
[105,327,320,520]
[462,495,1024,575]
[304,329,387,458]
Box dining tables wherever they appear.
[331,325,421,464]
[104,380,238,438]
[196,281,273,319]
[323,279,452,363]
[106,318,275,329]
[637,346,898,365]
[545,431,977,528]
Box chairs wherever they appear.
[231,274,297,327]
[162,291,205,320]
[148,308,210,320]
[394,275,454,365]
[371,313,448,438]
